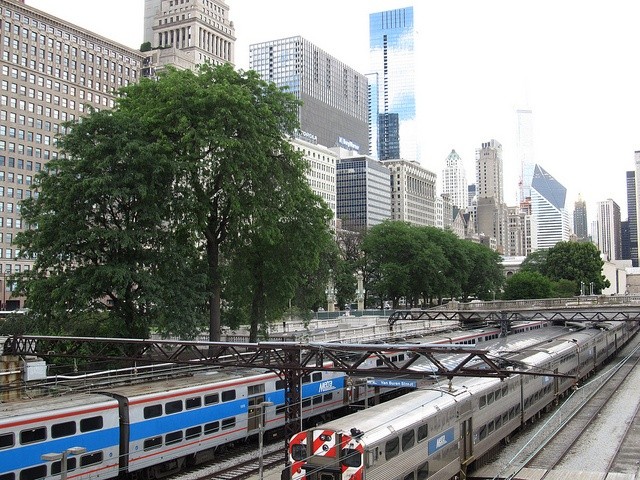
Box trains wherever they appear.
[287,320,640,480]
[0,320,556,480]
